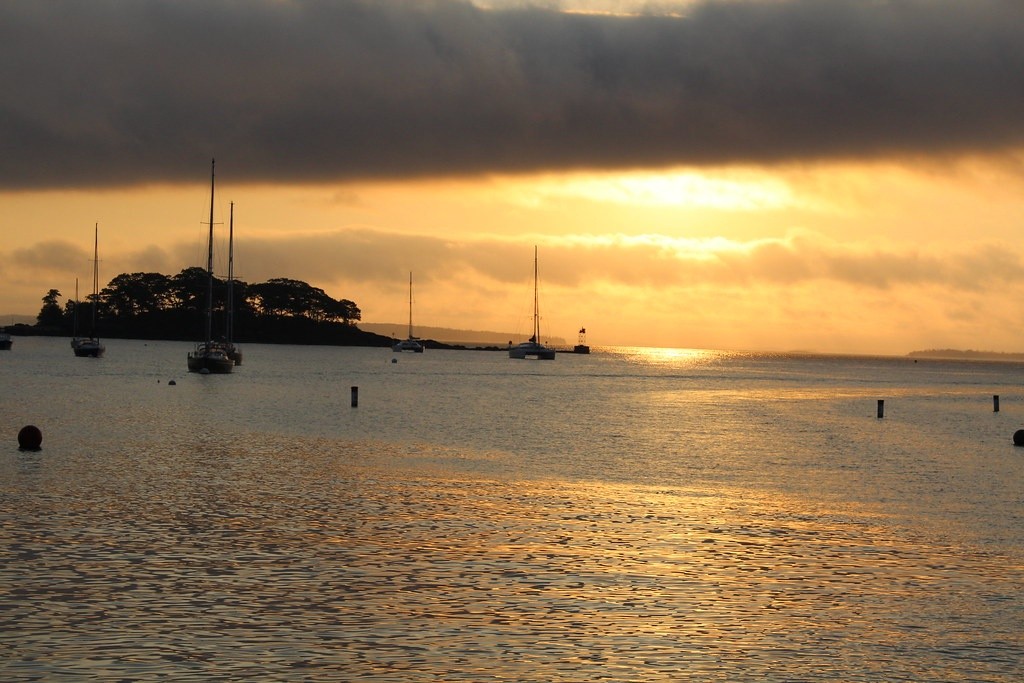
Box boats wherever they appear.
[1,334,13,350]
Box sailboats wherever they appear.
[200,201,243,366]
[509,245,555,359]
[187,157,235,374]
[69,223,107,357]
[391,271,424,353]
